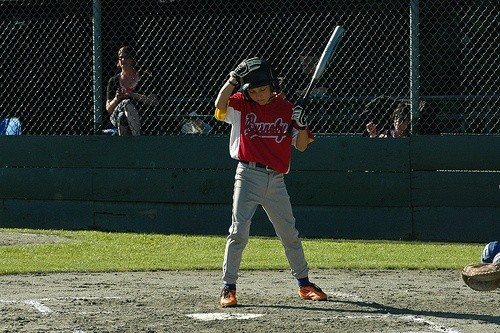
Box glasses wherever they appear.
[118,56,129,60]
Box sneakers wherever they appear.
[220,287,239,308]
[298,284,327,301]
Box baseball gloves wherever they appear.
[461,264,500,291]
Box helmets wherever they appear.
[237,58,280,92]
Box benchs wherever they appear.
[101,95,500,136]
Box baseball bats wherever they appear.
[285,26,345,137]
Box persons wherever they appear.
[106,47,158,136]
[215,57,327,307]
[463,241,500,291]
[366,108,408,138]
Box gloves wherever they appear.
[291,105,308,130]
[229,57,261,82]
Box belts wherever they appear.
[239,159,274,172]
